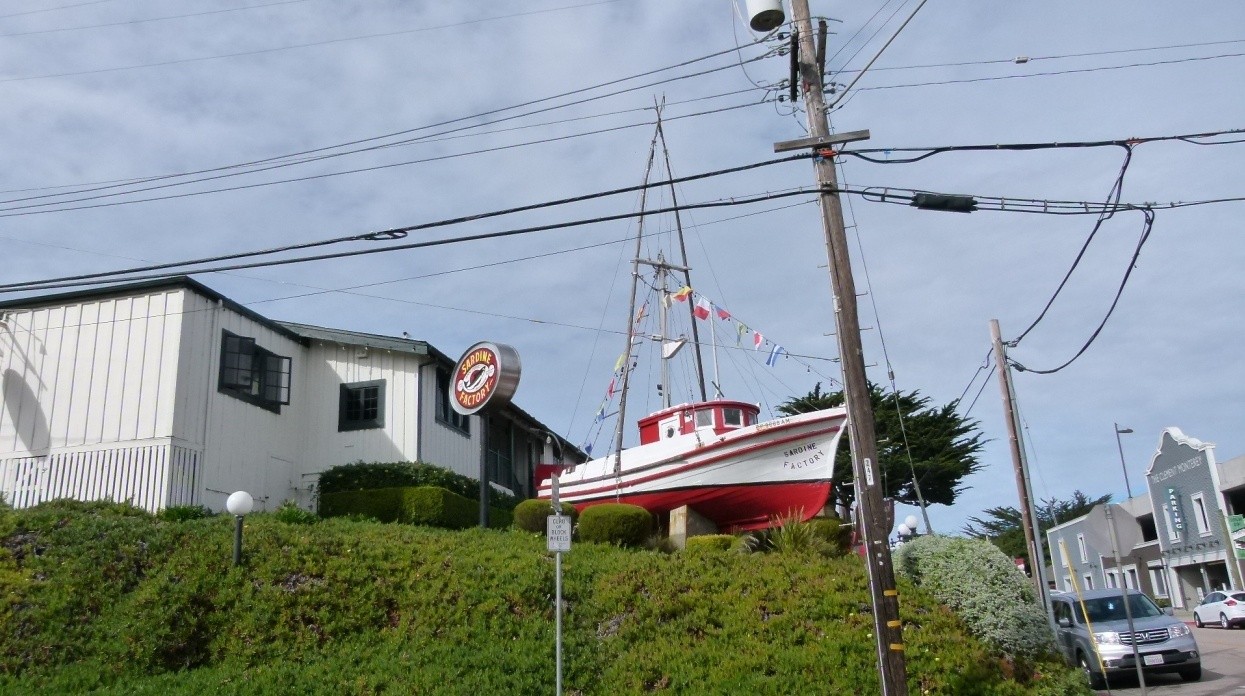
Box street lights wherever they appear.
[1114,422,1134,498]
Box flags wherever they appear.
[582,286,781,455]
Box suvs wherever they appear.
[1051,587,1201,691]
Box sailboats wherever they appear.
[532,90,849,537]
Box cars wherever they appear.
[1194,590,1245,629]
